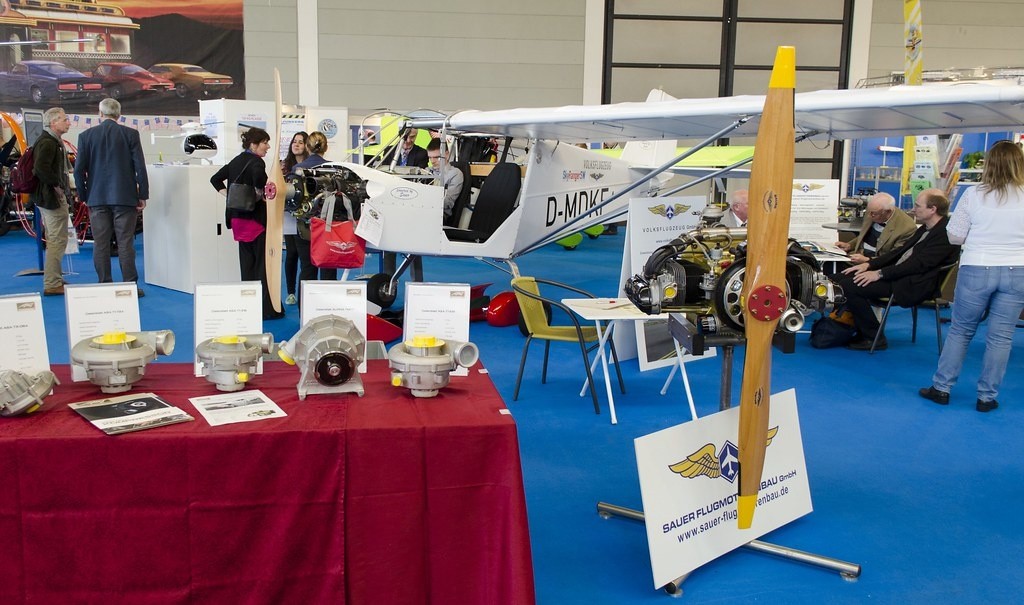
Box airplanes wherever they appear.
[264,67,1024,336]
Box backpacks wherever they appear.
[11,135,57,194]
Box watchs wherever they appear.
[877,269,882,278]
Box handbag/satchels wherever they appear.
[310,191,367,268]
[810,317,848,349]
[227,156,256,211]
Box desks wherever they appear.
[0,356,515,605]
[821,223,863,236]
[561,298,698,424]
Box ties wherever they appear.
[400,150,408,166]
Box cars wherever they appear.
[148,63,234,98]
[86,63,176,101]
[0,60,106,106]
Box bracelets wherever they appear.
[868,256,872,261]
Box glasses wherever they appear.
[428,156,445,161]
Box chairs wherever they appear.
[869,262,959,354]
[444,162,471,227]
[443,163,521,243]
[511,277,626,414]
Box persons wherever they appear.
[33,108,72,297]
[379,120,428,282]
[422,138,464,227]
[276,132,337,318]
[210,127,287,321]
[74,97,150,297]
[919,139,1024,413]
[363,131,381,169]
[822,189,961,352]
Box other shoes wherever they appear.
[44,281,70,296]
[848,330,888,350]
[138,289,144,297]
[919,386,949,404]
[976,398,999,412]
[263,310,285,320]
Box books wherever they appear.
[67,392,194,435]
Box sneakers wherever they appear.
[285,294,297,304]
